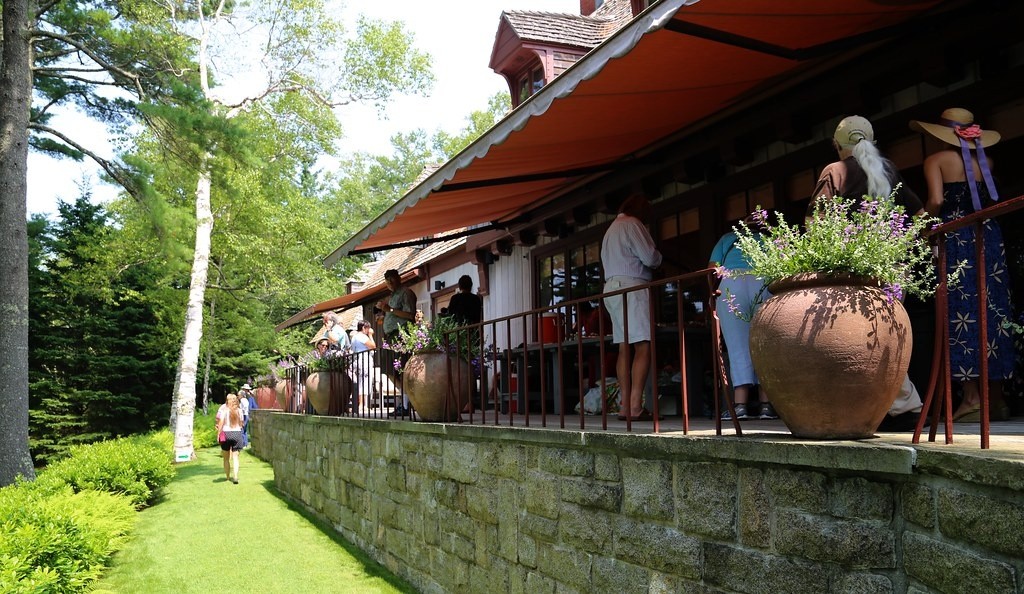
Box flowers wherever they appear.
[247,373,279,388]
[713,180,968,322]
[268,349,302,380]
[383,314,500,381]
[301,344,357,371]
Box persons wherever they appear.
[569,302,593,336]
[449,278,482,413]
[215,384,256,484]
[286,312,376,414]
[603,194,675,421]
[917,108,1015,424]
[711,215,778,421]
[381,269,417,416]
[805,117,930,431]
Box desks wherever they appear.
[485,325,715,416]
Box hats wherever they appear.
[315,336,329,349]
[909,108,1001,212]
[834,116,877,151]
[385,269,399,276]
[241,384,252,389]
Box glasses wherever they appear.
[321,345,329,347]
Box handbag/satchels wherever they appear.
[219,431,226,443]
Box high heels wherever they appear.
[991,407,1009,421]
[952,407,981,422]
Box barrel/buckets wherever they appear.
[538,313,565,344]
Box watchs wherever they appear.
[390,308,394,312]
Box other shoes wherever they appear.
[462,407,474,412]
[884,411,934,431]
[389,403,409,416]
[234,480,238,484]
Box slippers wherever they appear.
[618,416,626,420]
[631,408,664,421]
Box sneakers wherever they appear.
[759,402,778,419]
[721,404,749,419]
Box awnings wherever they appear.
[322,0,927,269]
[274,270,420,332]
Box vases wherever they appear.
[251,385,276,408]
[275,380,303,414]
[748,270,913,440]
[401,349,474,421]
[306,368,354,416]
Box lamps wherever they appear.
[434,280,445,290]
